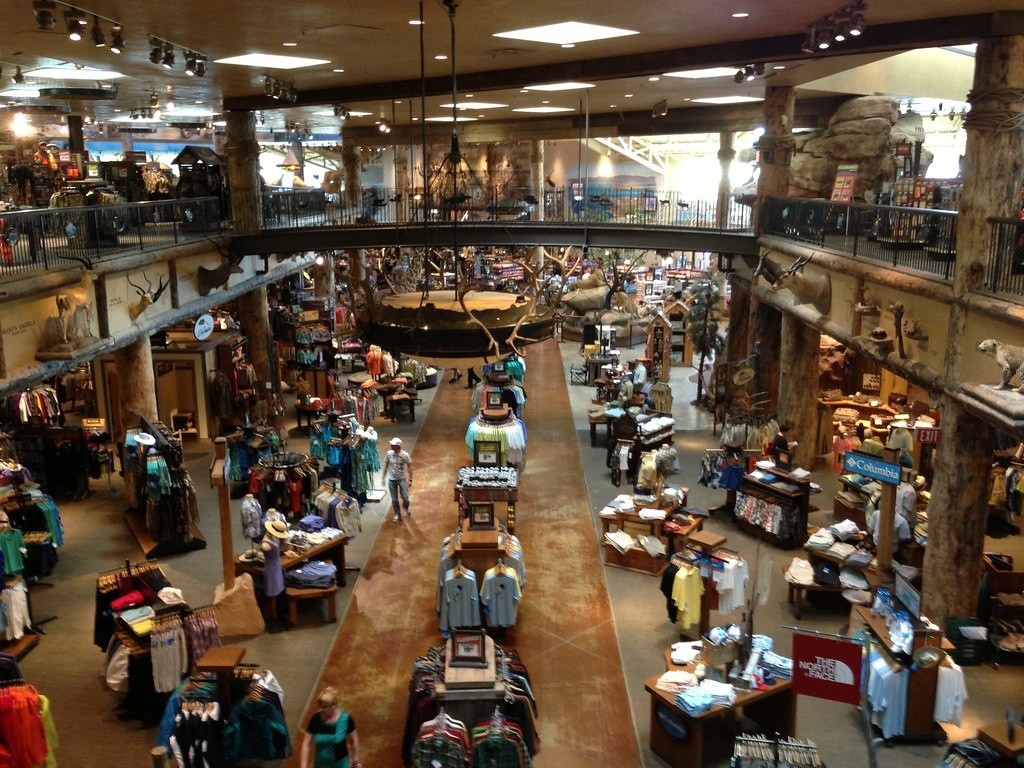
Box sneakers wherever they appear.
[392,506,412,522]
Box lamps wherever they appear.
[67,20,84,41]
[746,67,754,82]
[167,98,175,109]
[196,62,206,78]
[184,60,196,76]
[849,13,864,36]
[150,94,158,107]
[817,30,831,49]
[163,52,174,69]
[340,111,346,120]
[11,66,24,83]
[801,34,816,55]
[380,120,387,131]
[385,126,391,133]
[57,107,316,149]
[833,21,849,41]
[92,28,106,48]
[273,87,281,100]
[660,102,668,116]
[734,71,744,84]
[149,48,162,64]
[755,64,765,76]
[265,83,272,97]
[111,36,125,54]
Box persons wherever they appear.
[632,359,650,397]
[465,366,482,389]
[367,345,381,376]
[617,375,634,410]
[241,492,262,539]
[298,687,364,768]
[260,521,285,597]
[33,140,58,186]
[260,506,290,551]
[381,436,413,523]
[868,497,911,563]
[897,474,927,542]
[381,349,397,374]
[861,433,884,462]
[887,425,915,464]
[449,367,463,383]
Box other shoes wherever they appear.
[449,378,455,383]
[456,375,462,380]
[464,386,473,389]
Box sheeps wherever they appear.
[127,271,173,322]
[754,248,830,315]
[197,236,244,297]
[55,251,99,344]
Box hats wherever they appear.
[390,437,402,446]
[913,647,945,668]
[889,420,917,430]
[733,368,755,386]
[264,519,288,538]
[914,476,931,546]
[134,433,156,445]
[38,141,46,145]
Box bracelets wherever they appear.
[352,759,361,766]
[408,479,412,482]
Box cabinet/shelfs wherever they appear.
[0,212,1024,766]
